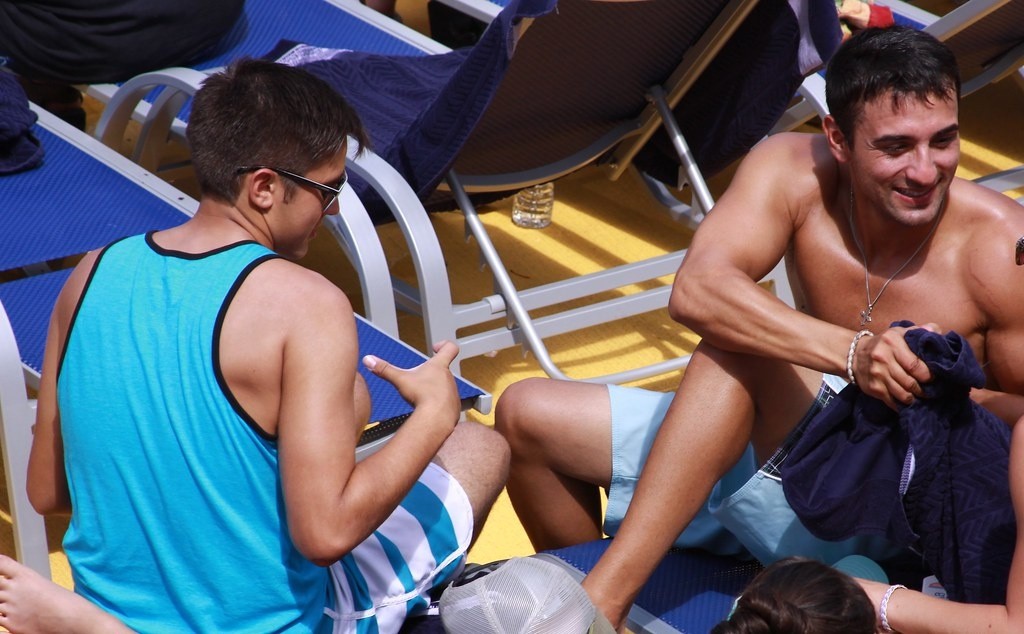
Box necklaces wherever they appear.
[851,177,944,326]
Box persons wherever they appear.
[490,26,1023,633]
[711,237,1024,634]
[26,56,512,634]
[0,0,244,176]
[0,555,140,634]
[837,0,894,43]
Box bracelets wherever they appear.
[879,585,907,633]
[848,330,874,387]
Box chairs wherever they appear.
[0,0,1024,634]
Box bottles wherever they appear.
[512,182,554,229]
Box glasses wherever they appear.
[235,163,350,213]
